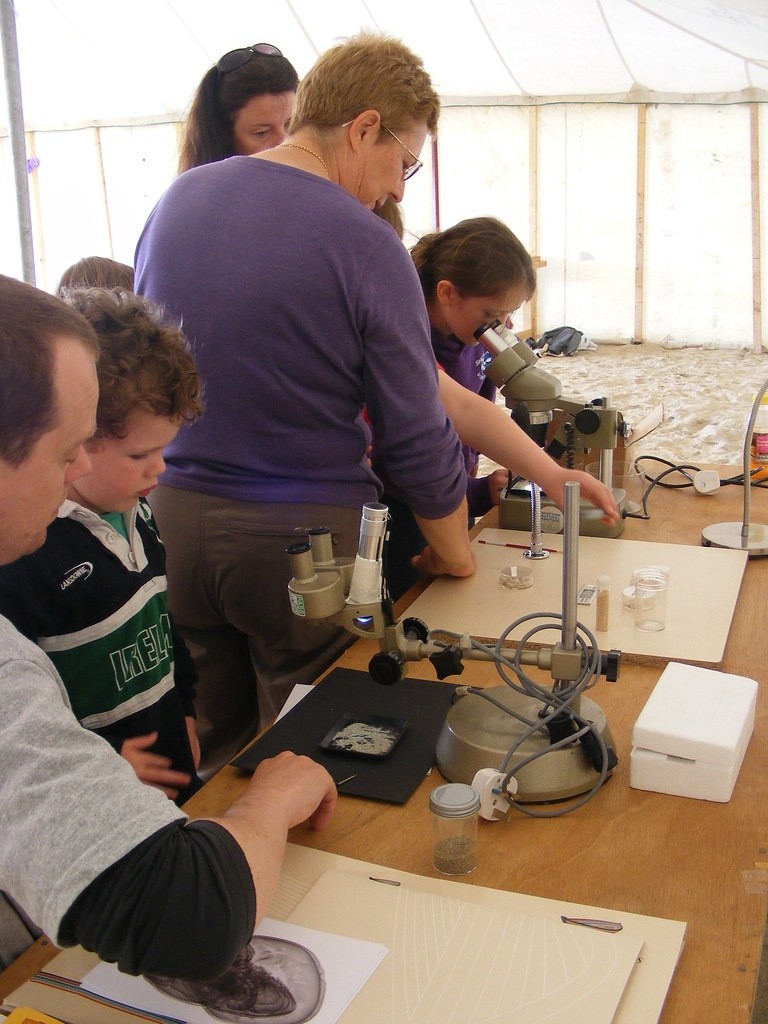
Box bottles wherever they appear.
[633,571,668,630]
[429,784,480,874]
[742,395,768,478]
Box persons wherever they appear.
[0,285,206,810]
[54,41,619,586]
[0,270,338,985]
[133,25,477,769]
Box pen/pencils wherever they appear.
[478,539,565,554]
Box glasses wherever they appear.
[217,43,283,78]
[342,111,424,181]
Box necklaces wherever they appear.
[279,142,332,177]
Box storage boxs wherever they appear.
[628,661,760,804]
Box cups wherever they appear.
[586,461,646,514]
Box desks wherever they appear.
[0,459,768,1024]
